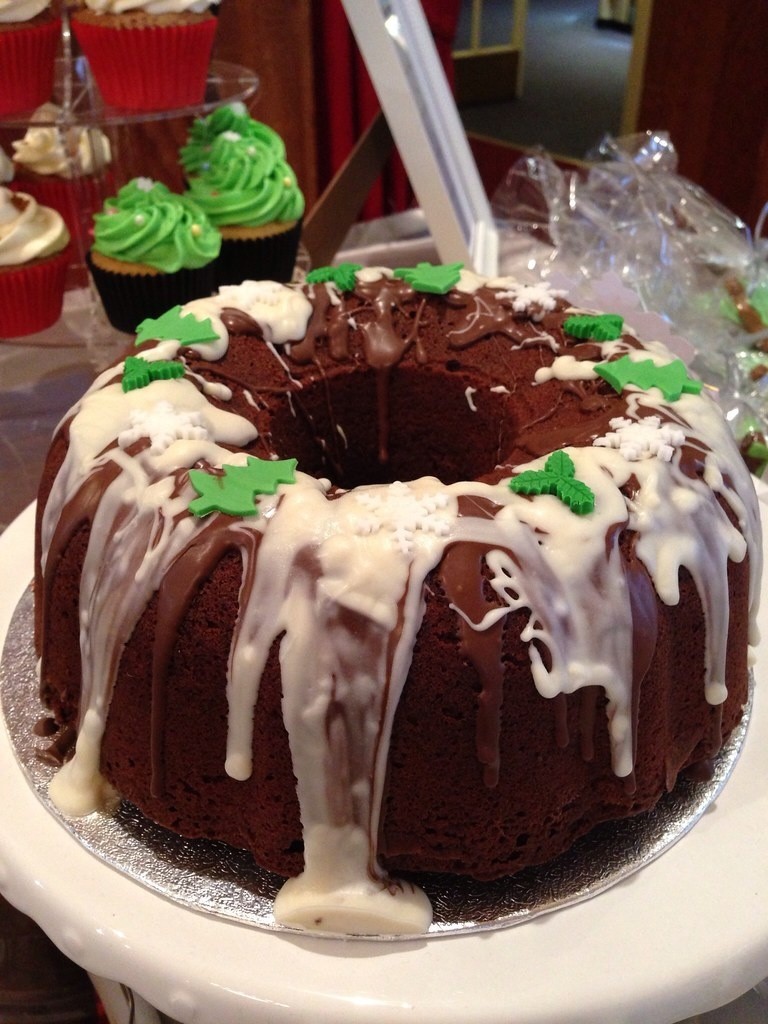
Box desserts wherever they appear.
[0,0,307,336]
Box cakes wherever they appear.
[32,262,762,943]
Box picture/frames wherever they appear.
[300,0,499,278]
